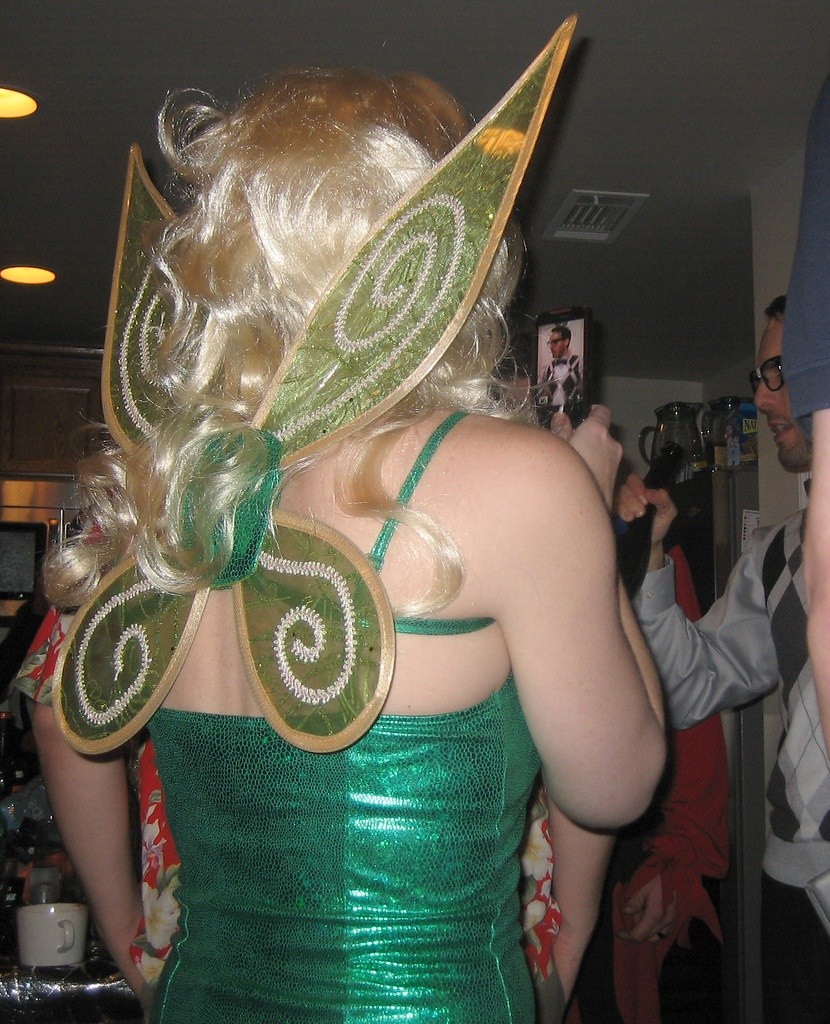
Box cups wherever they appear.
[28,866,60,905]
[16,903,88,965]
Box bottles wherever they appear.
[3,786,25,832]
[612,442,685,603]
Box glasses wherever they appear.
[547,337,564,344]
[748,357,784,390]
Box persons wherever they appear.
[28,72,830,1024]
[538,327,584,408]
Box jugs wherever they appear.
[638,401,701,463]
[700,397,746,452]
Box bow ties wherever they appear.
[554,357,568,366]
